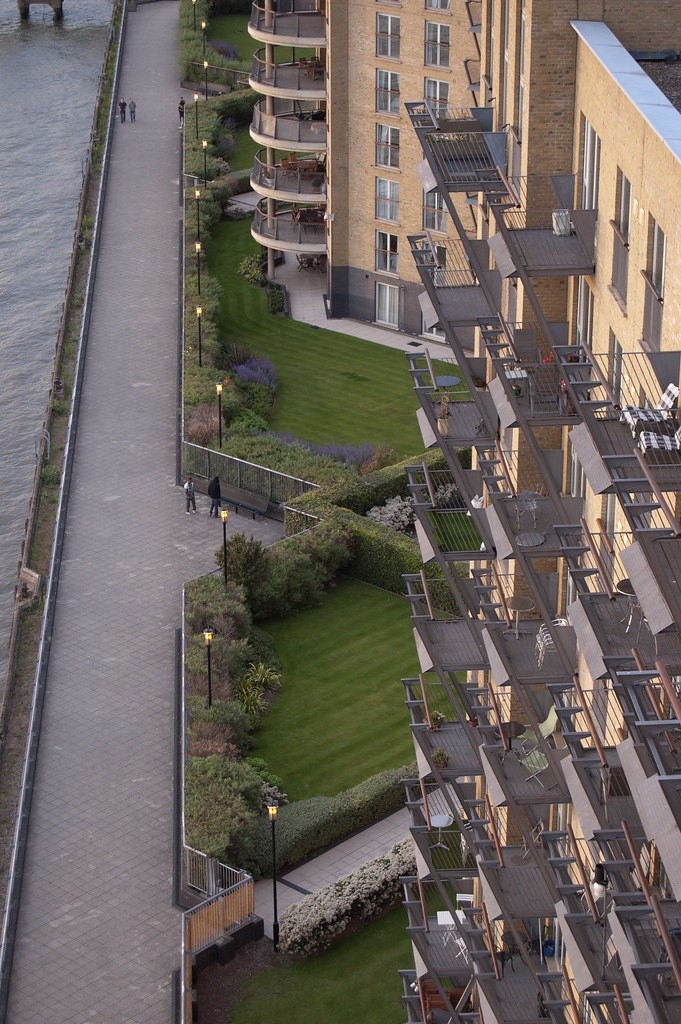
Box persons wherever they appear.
[183,477,197,515]
[207,476,222,518]
[128,97,136,123]
[118,97,127,124]
[177,96,186,131]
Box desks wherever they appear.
[494,720,526,768]
[615,578,652,635]
[430,374,463,413]
[501,930,530,967]
[501,596,537,640]
[427,814,454,850]
[436,910,463,949]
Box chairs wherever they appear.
[454,936,470,965]
[516,704,560,756]
[488,944,516,979]
[291,206,325,235]
[513,481,545,531]
[581,842,652,920]
[298,56,326,79]
[296,108,328,135]
[520,817,545,860]
[281,151,316,177]
[296,253,327,274]
[455,893,475,925]
[527,925,550,966]
[636,425,681,459]
[534,618,570,671]
[618,382,680,438]
[517,743,569,792]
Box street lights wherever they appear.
[200,20,206,62]
[192,92,201,141]
[190,0,197,33]
[192,240,202,300]
[214,381,224,453]
[201,60,209,102]
[200,139,207,190]
[193,189,200,244]
[262,797,286,953]
[201,625,215,711]
[218,508,230,591]
[194,305,204,368]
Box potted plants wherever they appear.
[423,711,446,733]
[311,178,322,193]
[431,747,450,769]
